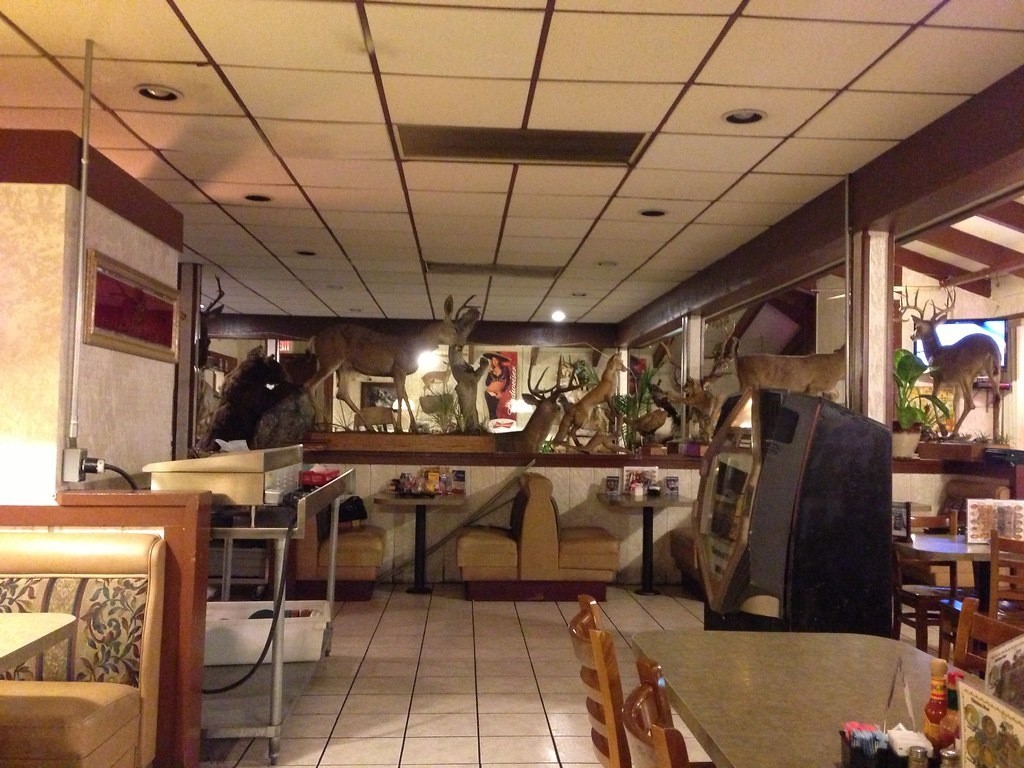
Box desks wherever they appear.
[892,534,1024,680]
[894,502,932,517]
[374,491,466,595]
[631,630,1024,768]
[0,613,76,675]
[597,493,699,597]
[155,466,356,767]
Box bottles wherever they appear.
[906,660,968,768]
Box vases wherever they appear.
[281,352,317,381]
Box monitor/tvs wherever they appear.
[913,319,1008,369]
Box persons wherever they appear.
[481,350,512,420]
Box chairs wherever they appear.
[909,509,958,535]
[893,551,973,654]
[953,598,1024,679]
[621,656,689,768]
[567,594,716,768]
[987,530,1024,656]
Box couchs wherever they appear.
[937,481,1013,533]
[533,472,620,602]
[296,510,387,600]
[457,474,538,601]
[0,531,167,768]
[670,528,703,601]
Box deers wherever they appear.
[893,285,1001,442]
[737,306,909,396]
[422,360,452,394]
[490,354,590,452]
[303,295,480,432]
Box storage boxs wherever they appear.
[204,601,330,666]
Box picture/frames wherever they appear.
[83,247,181,364]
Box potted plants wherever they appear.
[894,350,951,459]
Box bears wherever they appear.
[198,344,291,453]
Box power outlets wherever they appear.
[61,448,88,482]
